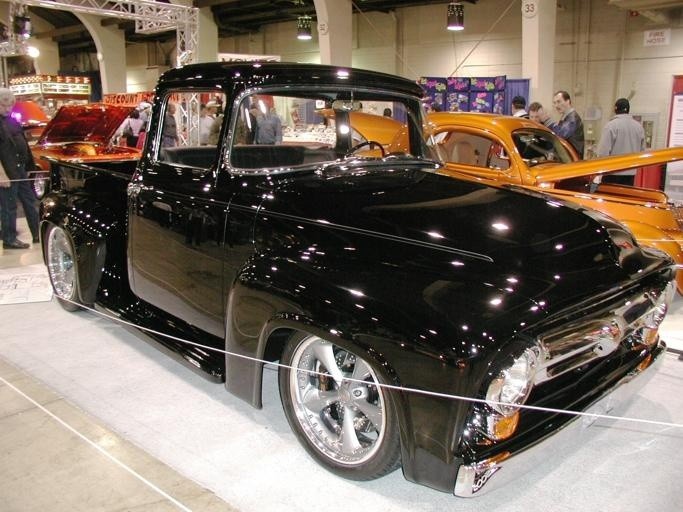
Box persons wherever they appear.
[592,98,645,189]
[500,95,530,169]
[0,87,39,249]
[383,108,391,119]
[109,93,282,151]
[428,104,447,146]
[537,90,584,161]
[522,102,553,159]
[351,103,369,149]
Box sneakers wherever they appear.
[32,232,41,243]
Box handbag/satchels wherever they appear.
[121,125,133,138]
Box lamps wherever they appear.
[446,0,465,31]
[13,4,32,34]
[180,50,192,65]
[296,0,313,41]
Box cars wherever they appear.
[31,60,680,497]
[9,101,143,199]
[315,105,682,293]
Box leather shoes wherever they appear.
[2,237,30,250]
